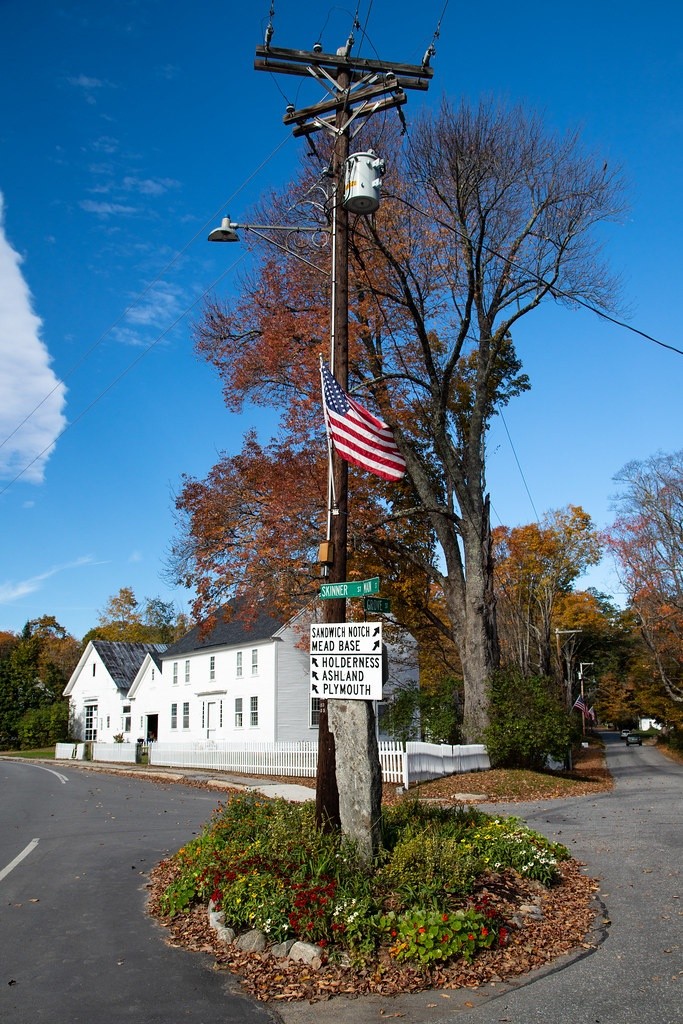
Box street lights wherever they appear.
[206,180,350,833]
[578,662,594,736]
[556,627,583,770]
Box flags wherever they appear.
[589,708,595,720]
[574,697,585,712]
[320,360,408,481]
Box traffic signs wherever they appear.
[309,623,383,656]
[363,596,391,614]
[319,577,379,600]
[308,654,383,701]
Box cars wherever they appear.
[625,733,642,746]
[620,730,631,740]
